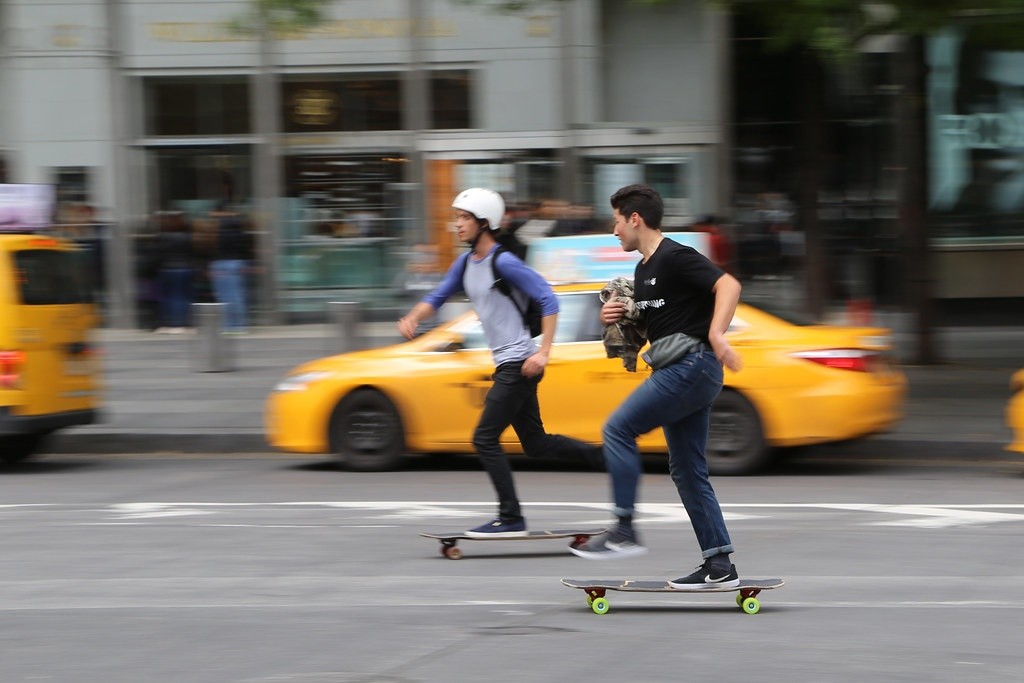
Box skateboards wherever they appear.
[560,577,787,615]
[417,526,609,560]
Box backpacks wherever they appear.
[460,253,543,338]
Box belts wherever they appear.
[687,342,713,353]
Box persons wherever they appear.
[571,185,742,590]
[395,189,604,538]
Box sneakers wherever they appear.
[667,558,740,591]
[465,516,528,537]
[565,523,648,559]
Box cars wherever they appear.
[261,274,906,472]
[2,230,105,465]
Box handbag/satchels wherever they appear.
[641,333,700,370]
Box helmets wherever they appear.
[452,188,506,230]
